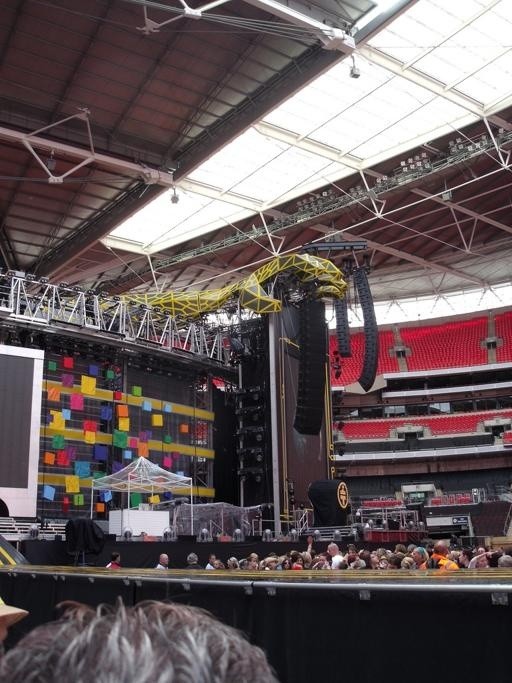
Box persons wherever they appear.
[105,551,122,568]
[156,553,170,569]
[0,594,282,682]
[0,595,35,656]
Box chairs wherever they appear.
[328,309,512,441]
[363,492,473,508]
[154,322,234,389]
